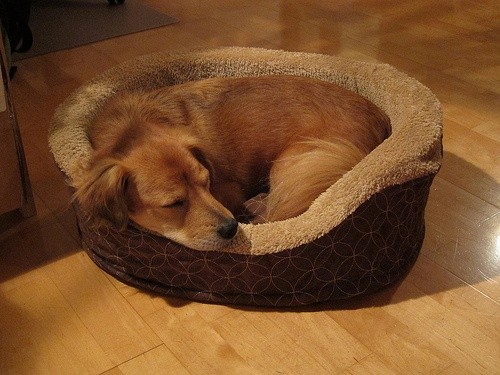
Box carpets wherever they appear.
[0,0,181,63]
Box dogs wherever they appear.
[62,75,393,252]
